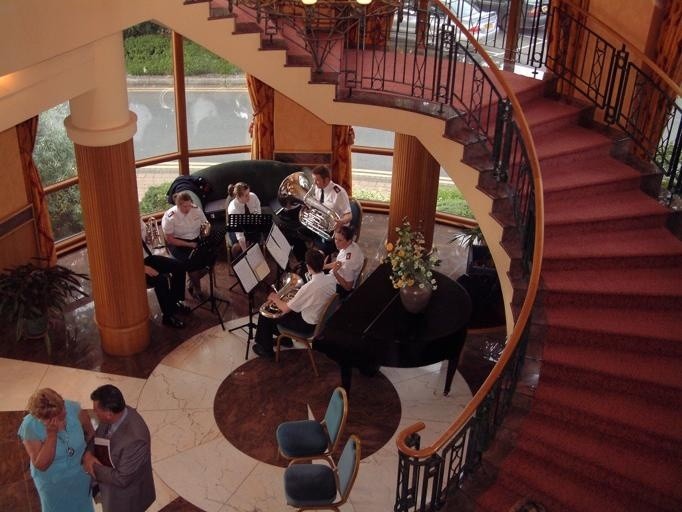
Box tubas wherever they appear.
[278,171,342,240]
[259,272,305,318]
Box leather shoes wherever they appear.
[176,301,192,317]
[273,335,293,349]
[252,343,277,361]
[196,291,208,304]
[161,313,185,329]
[187,278,196,296]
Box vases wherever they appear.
[397,283,434,314]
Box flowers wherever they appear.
[382,222,445,292]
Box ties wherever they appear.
[241,204,254,214]
[319,189,326,204]
[142,238,154,257]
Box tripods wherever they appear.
[228,292,259,360]
[189,261,229,331]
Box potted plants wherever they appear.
[447,226,502,300]
[0,260,90,343]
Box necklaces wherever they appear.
[54,423,74,457]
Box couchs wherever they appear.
[167,159,316,241]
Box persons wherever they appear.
[164,173,212,211]
[141,219,195,329]
[161,192,212,305]
[82,384,156,512]
[227,165,363,365]
[17,387,97,512]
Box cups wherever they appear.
[480,336,497,359]
[490,339,503,360]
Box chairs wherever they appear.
[267,386,350,470]
[326,196,364,264]
[224,193,266,275]
[282,433,363,512]
[274,292,340,377]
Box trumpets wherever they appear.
[143,217,162,248]
